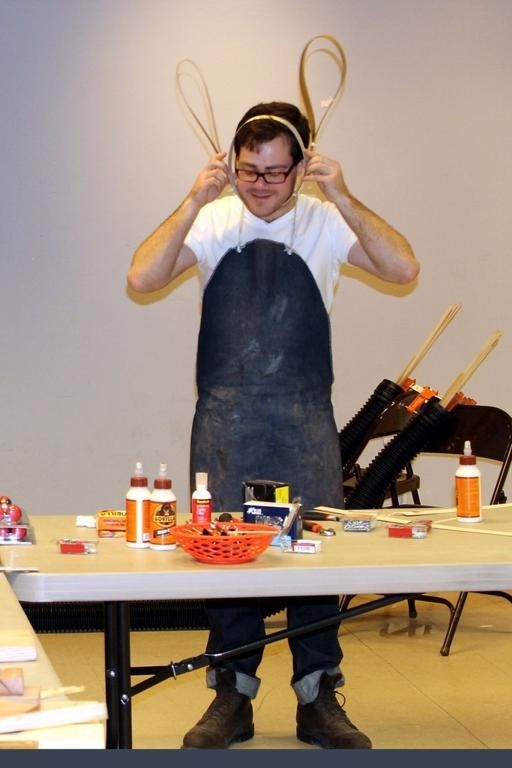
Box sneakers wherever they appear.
[296,671,371,749]
[182,669,255,749]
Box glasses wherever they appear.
[234,154,296,185]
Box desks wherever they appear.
[0,503,512,749]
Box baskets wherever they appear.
[171,522,279,564]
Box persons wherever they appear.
[125,101,420,749]
